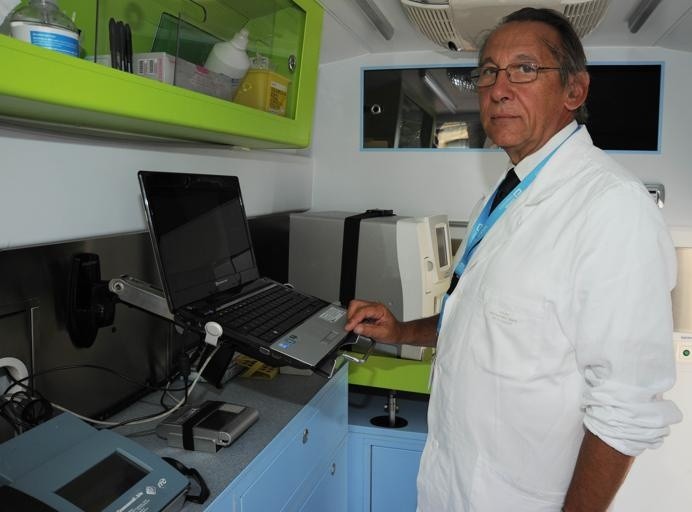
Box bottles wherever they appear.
[7,1,81,55]
[203,26,251,102]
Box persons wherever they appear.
[345,5,685,512]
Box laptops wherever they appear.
[137,170,369,370]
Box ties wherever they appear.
[488,167,521,215]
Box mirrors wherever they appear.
[359,64,668,152]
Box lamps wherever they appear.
[419,68,459,115]
[628,0,662,33]
[354,0,397,42]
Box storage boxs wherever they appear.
[1,0,325,151]
[76,51,234,104]
[232,65,292,118]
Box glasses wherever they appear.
[470,62,561,88]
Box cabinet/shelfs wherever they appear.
[343,429,429,510]
[364,77,438,148]
[225,368,349,512]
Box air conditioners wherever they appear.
[400,0,611,54]
